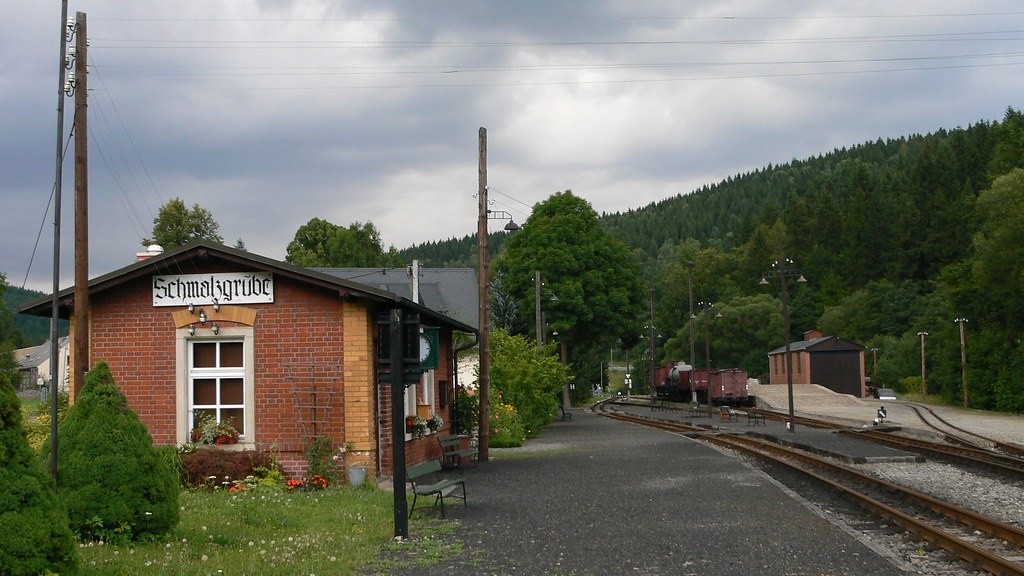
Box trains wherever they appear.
[654,362,748,407]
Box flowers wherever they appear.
[406,415,428,439]
[430,414,444,432]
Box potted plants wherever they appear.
[188,407,239,450]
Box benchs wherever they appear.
[648,398,678,414]
[559,406,572,421]
[686,402,712,417]
[437,434,480,470]
[405,459,467,519]
[720,408,739,422]
[746,409,765,427]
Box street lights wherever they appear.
[478,211,520,461]
[690,301,723,417]
[758,269,807,431]
[536,292,559,345]
[640,325,663,410]
[541,322,558,350]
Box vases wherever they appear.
[426,419,433,426]
[406,423,414,431]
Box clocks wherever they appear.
[420,332,432,364]
